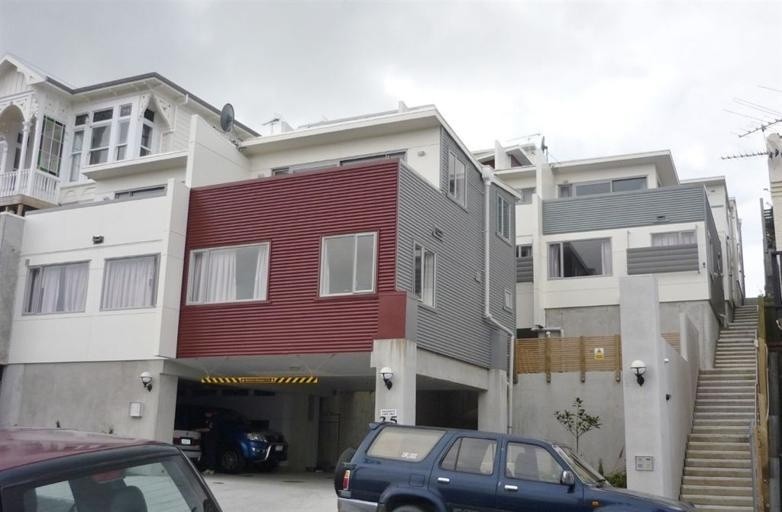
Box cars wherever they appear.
[2,427,221,511]
[176,407,286,474]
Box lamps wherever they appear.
[629,359,647,386]
[140,372,153,392]
[378,367,393,390]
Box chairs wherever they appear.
[110,481,148,511]
[514,453,536,480]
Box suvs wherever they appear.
[334,423,695,512]
